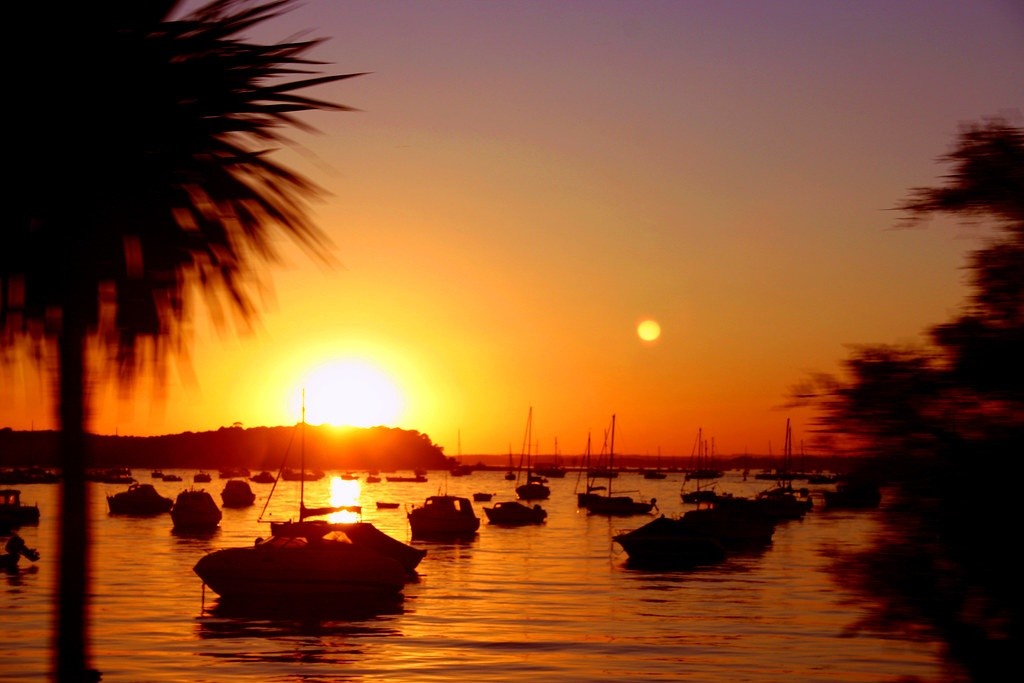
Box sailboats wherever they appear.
[483,403,846,578]
[448,428,473,477]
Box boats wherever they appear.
[406,493,482,538]
[1,383,429,614]
[472,492,492,502]
